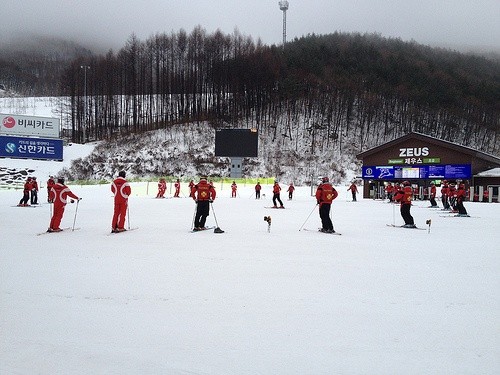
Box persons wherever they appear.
[48,176,80,232]
[31,176,39,205]
[255,182,261,199]
[347,183,359,201]
[286,182,295,200]
[272,179,284,208]
[210,180,213,186]
[231,181,237,198]
[192,177,216,231]
[441,179,467,215]
[189,180,195,197]
[47,176,55,203]
[383,181,401,204]
[111,171,131,231]
[316,177,338,233]
[173,179,181,197]
[429,181,437,206]
[156,178,167,198]
[18,177,35,207]
[392,180,416,228]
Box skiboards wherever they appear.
[303,227,342,235]
[189,225,216,233]
[439,214,479,218]
[9,202,43,208]
[263,205,287,210]
[108,226,139,234]
[386,223,428,230]
[36,227,81,236]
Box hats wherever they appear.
[443,180,447,184]
[119,170,126,177]
[456,178,463,182]
[322,177,329,183]
[58,176,64,183]
[200,175,208,180]
[430,181,435,184]
[402,180,411,186]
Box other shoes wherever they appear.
[279,205,284,208]
[202,227,206,229]
[53,228,61,231]
[321,228,325,232]
[405,224,414,227]
[272,205,277,208]
[194,227,201,230]
[325,229,334,233]
[112,229,117,232]
[118,228,126,231]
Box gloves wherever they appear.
[317,201,320,204]
[209,200,213,203]
[195,199,198,203]
